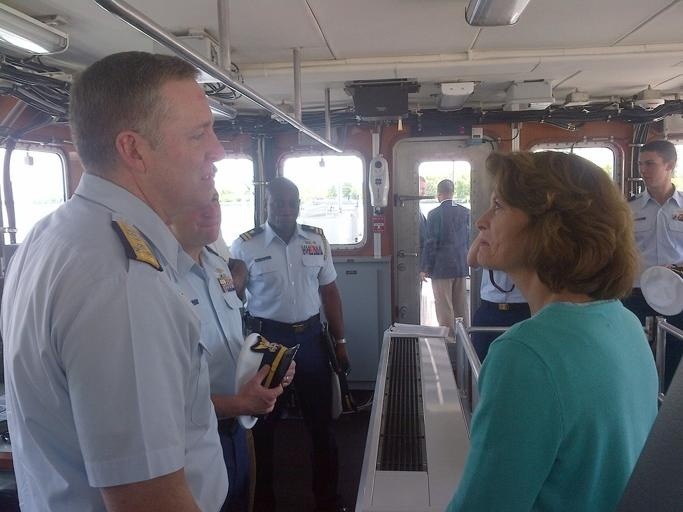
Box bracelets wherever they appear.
[334,335,347,346]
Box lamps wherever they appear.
[204,95,238,121]
[0,3,69,56]
[436,82,474,111]
[564,88,592,107]
[464,0,529,27]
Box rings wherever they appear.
[285,376,288,382]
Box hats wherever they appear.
[234,333,299,431]
[330,368,358,421]
[639,266,682,316]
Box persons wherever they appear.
[465,229,531,363]
[619,139,682,407]
[165,185,298,512]
[427,179,470,342]
[416,176,427,290]
[0,52,231,511]
[228,177,351,511]
[443,150,660,510]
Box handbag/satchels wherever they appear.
[323,323,339,373]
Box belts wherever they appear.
[479,299,529,311]
[261,316,320,333]
